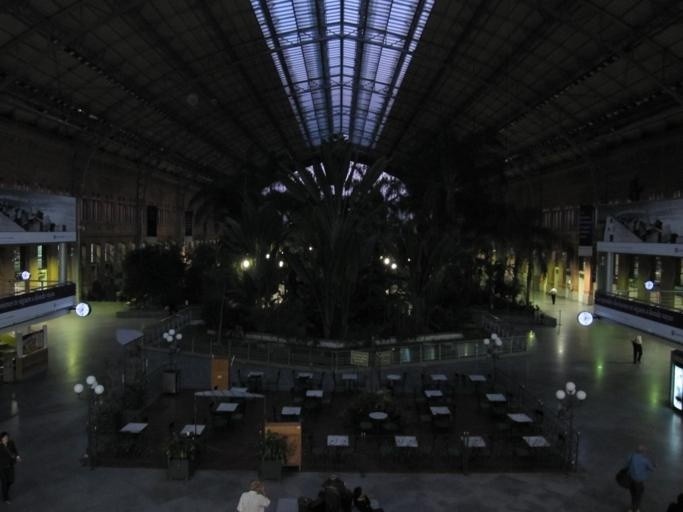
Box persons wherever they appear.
[0,431,25,505]
[628,444,658,512]
[236,478,271,512]
[550,288,557,304]
[632,335,643,364]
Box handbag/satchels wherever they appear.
[614,466,637,489]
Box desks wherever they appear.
[118,370,551,471]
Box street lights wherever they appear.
[483,332,504,381]
[555,380,585,463]
[73,375,106,468]
[161,327,184,371]
